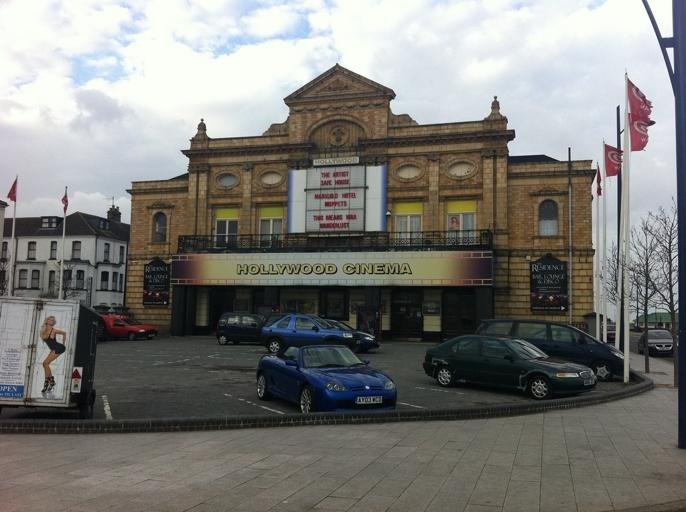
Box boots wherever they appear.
[41,376,56,394]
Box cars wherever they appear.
[602,325,616,343]
[423,334,598,400]
[638,330,679,357]
[216,312,397,413]
[99,314,157,341]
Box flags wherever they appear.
[626,78,651,122]
[602,145,625,177]
[7,178,17,202]
[630,115,658,152]
[596,168,602,196]
[61,195,69,215]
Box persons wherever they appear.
[39,315,68,394]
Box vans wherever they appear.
[467,318,624,382]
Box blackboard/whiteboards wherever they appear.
[143,259,170,307]
[529,255,570,314]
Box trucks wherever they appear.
[0,296,96,418]
